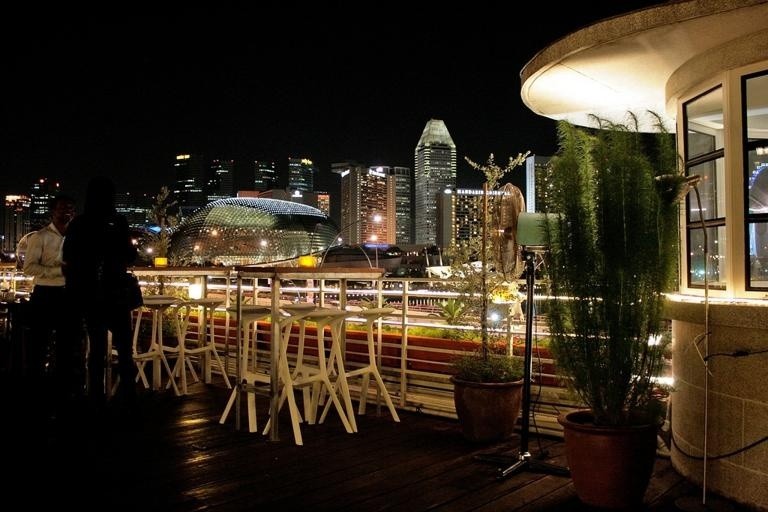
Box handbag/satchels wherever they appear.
[112,275,144,315]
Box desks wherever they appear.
[234,264,386,440]
[104,265,232,402]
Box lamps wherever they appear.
[652,173,734,512]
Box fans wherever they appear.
[469,183,570,481]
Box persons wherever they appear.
[61,169,143,403]
[16,192,88,389]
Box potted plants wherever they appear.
[535,110,686,509]
[428,151,532,441]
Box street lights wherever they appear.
[316,208,382,283]
